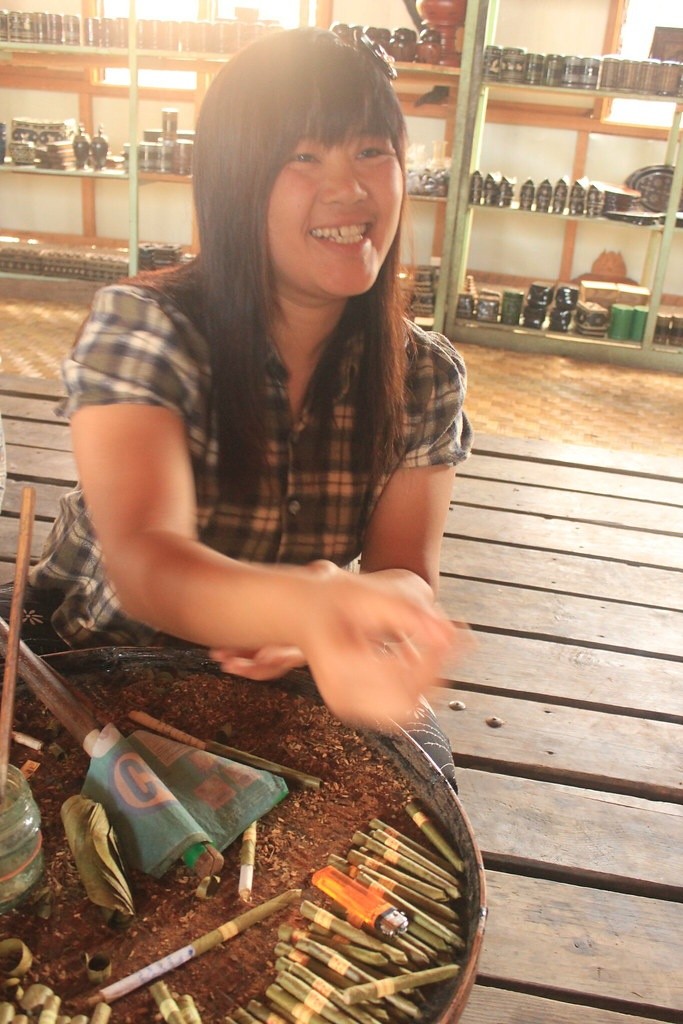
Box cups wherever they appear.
[140,139,193,175]
[159,108,179,144]
[0,763,44,909]
[410,268,437,316]
[454,294,473,317]
[477,297,499,322]
[502,289,524,325]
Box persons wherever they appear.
[15,27,468,797]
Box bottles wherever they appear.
[122,143,130,171]
[90,130,108,170]
[0,122,7,162]
[72,127,88,170]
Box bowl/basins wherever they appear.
[7,141,35,164]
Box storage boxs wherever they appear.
[11,116,76,150]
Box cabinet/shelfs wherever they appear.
[445,0,683,373]
[0,0,480,333]
